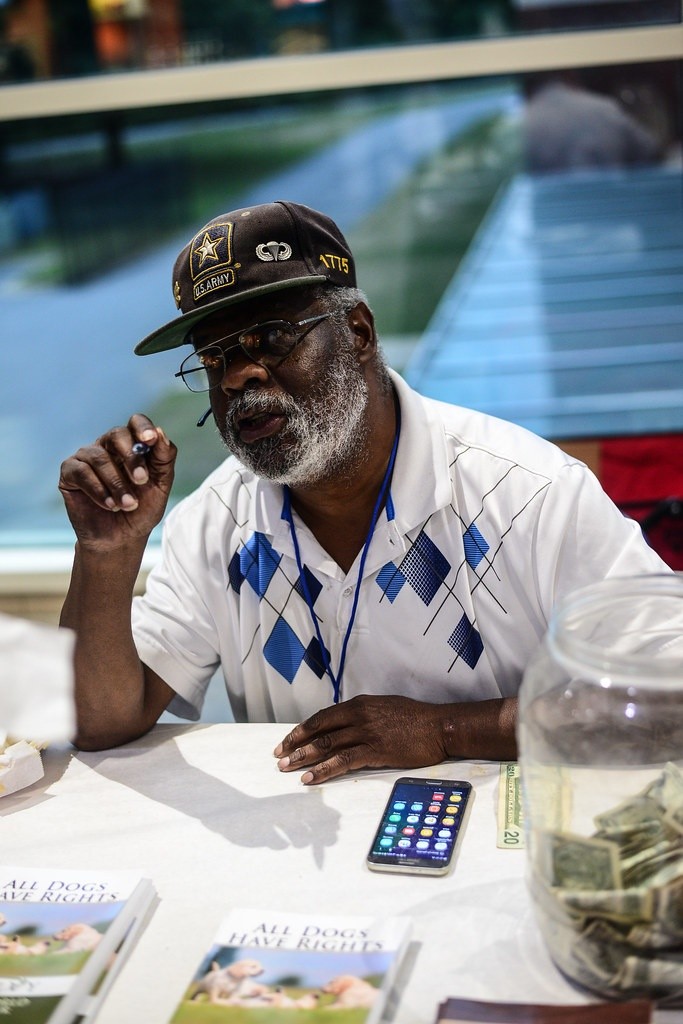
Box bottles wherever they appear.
[516,573,683,1012]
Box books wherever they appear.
[172,906,423,1024]
[0,865,162,1024]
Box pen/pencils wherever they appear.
[127,443,150,455]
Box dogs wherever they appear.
[192,957,319,1009]
[320,974,377,1010]
[0,913,104,955]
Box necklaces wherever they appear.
[282,365,400,703]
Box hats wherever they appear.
[134,203,356,358]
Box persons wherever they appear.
[56,201,673,786]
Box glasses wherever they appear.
[173,310,334,395]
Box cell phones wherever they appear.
[367,777,472,876]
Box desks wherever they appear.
[0,722,683,1024]
